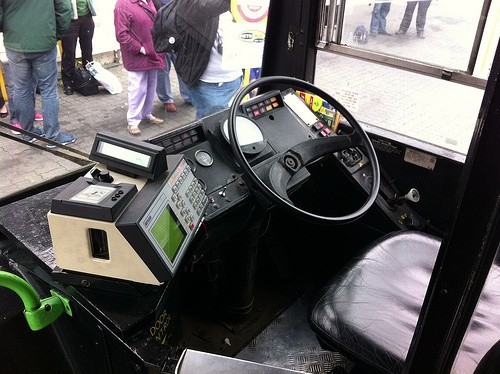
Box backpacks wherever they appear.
[150,0,189,53]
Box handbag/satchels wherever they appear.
[61,52,99,95]
[85,57,124,93]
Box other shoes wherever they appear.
[163,99,177,112]
[46,132,77,148]
[0,104,8,118]
[11,122,24,135]
[184,100,193,106]
[396,29,406,34]
[128,124,142,135]
[379,30,393,36]
[63,79,73,95]
[369,31,378,37]
[142,113,163,125]
[417,29,426,39]
[34,111,43,120]
[22,126,45,142]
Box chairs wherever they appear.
[307,228,500,374]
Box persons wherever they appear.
[173,0,244,120]
[395,0,432,39]
[369,0,393,38]
[0,0,77,148]
[114,0,194,135]
[61,0,96,94]
